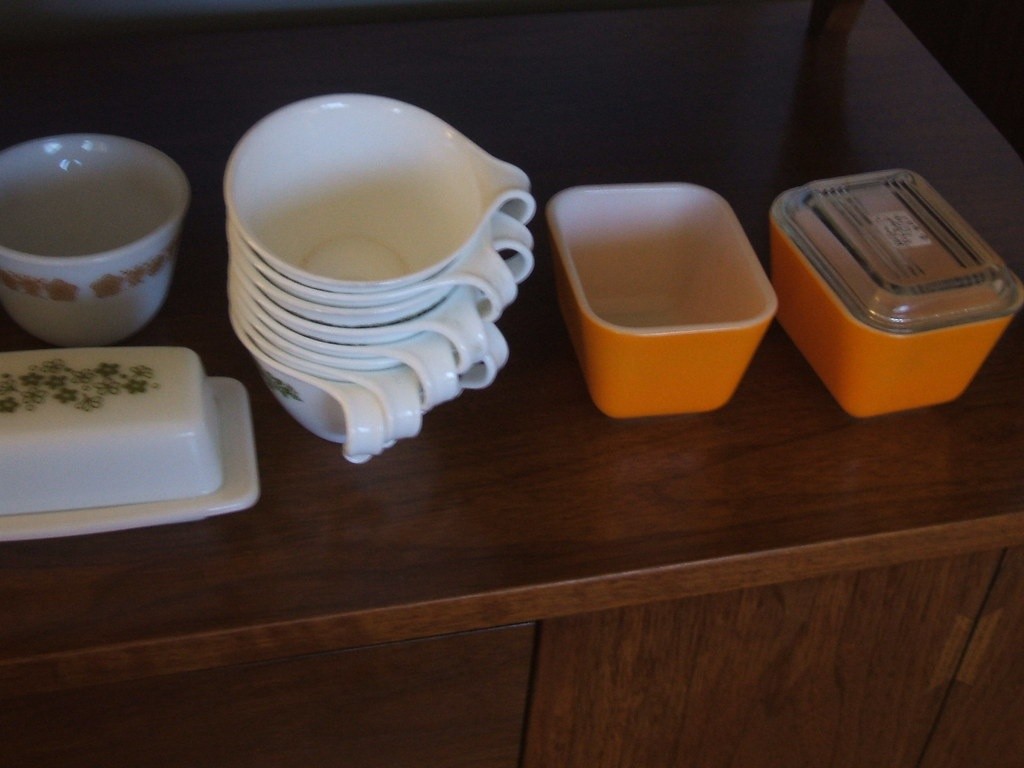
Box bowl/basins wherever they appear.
[771,168,1023,419]
[547,183,779,419]
[0,348,224,515]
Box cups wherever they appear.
[1,132,191,347]
[224,93,537,462]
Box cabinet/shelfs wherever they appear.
[0,0,1021,767]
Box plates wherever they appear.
[0,376,259,542]
